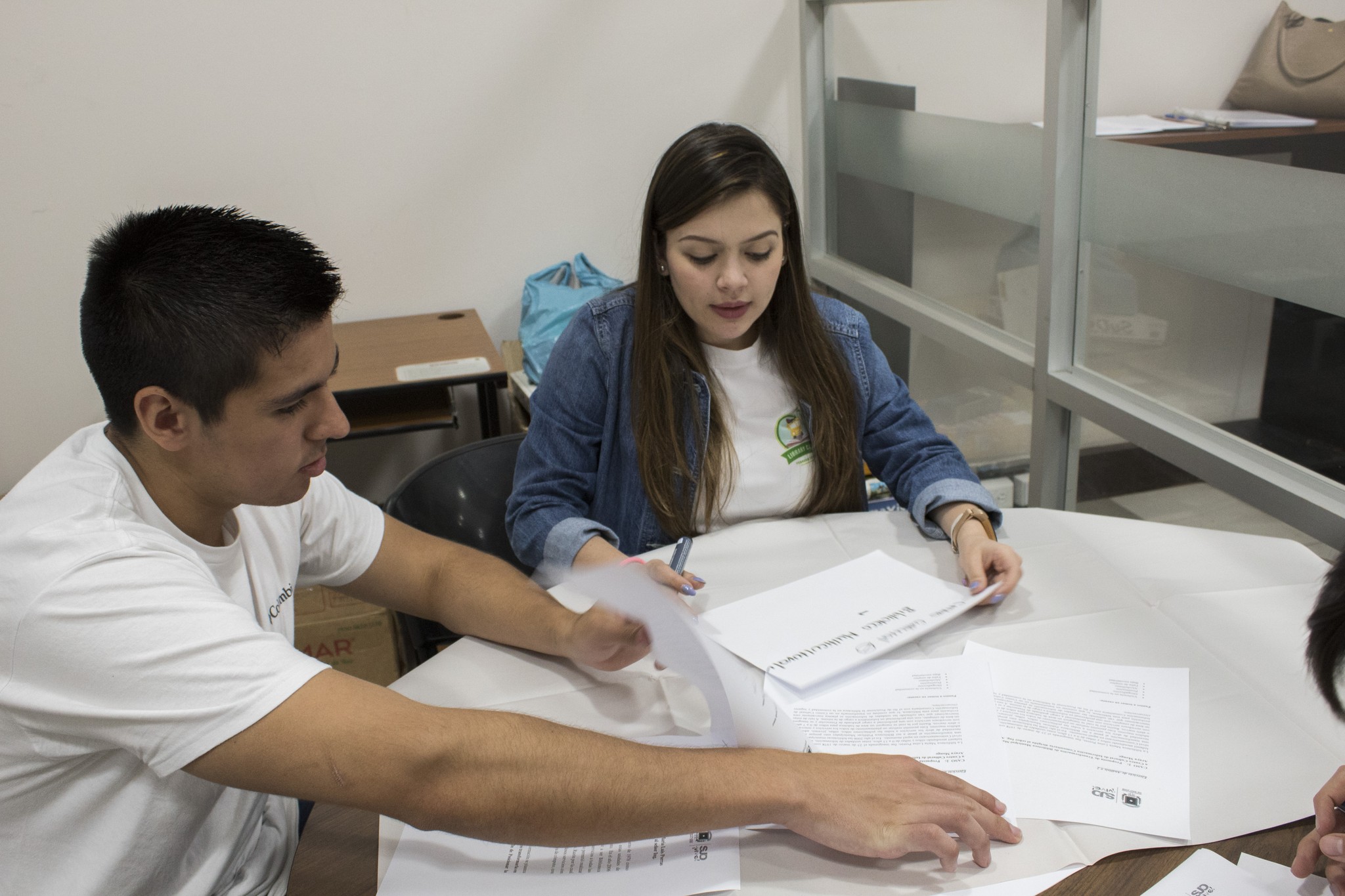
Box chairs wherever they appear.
[384,429,529,660]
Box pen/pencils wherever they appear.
[1164,112,1188,120]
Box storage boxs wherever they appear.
[292,585,404,687]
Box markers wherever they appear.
[669,535,693,577]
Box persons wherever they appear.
[0,206,1022,896]
[1290,549,1345,896]
[504,122,1022,616]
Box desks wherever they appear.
[323,308,545,443]
[285,508,1345,896]
[835,78,1345,486]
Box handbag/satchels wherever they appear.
[1221,0,1345,120]
[521,254,625,387]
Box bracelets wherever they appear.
[951,508,998,556]
